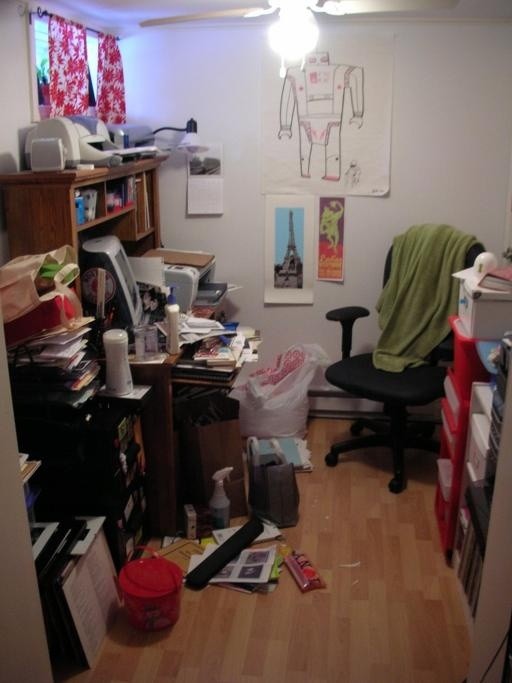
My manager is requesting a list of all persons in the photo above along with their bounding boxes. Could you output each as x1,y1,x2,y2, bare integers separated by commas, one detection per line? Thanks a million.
139,290,162,327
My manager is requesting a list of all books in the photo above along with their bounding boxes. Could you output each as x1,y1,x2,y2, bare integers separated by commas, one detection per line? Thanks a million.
135,172,150,233
174,317,265,381
18,453,41,485
476,265,512,291
216,583,263,593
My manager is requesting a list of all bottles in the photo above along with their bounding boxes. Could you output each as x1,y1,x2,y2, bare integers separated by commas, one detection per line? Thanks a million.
113,127,130,149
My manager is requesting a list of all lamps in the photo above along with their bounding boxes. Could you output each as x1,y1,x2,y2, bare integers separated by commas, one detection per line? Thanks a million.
146,114,212,158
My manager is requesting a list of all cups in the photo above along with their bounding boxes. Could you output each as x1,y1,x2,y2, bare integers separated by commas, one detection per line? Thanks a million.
101,328,133,397
74,196,85,226
133,324,159,362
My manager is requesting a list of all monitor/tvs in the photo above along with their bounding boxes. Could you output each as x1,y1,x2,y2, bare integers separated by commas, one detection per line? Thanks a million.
82,235,143,343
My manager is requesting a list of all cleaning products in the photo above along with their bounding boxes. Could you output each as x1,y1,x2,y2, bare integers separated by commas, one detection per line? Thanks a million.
208,466,236,530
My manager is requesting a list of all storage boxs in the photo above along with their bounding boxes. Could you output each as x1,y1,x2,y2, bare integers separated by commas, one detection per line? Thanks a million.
430,250,510,620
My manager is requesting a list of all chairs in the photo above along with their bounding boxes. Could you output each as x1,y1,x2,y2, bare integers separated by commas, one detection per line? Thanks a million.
319,222,488,497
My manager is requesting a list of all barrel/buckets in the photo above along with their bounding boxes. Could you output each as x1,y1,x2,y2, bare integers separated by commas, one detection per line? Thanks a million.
116,557,183,634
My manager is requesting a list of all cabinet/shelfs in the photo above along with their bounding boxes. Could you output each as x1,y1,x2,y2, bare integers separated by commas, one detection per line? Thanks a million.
1,143,273,579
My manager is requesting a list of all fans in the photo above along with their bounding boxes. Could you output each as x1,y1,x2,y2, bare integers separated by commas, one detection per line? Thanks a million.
133,0,466,32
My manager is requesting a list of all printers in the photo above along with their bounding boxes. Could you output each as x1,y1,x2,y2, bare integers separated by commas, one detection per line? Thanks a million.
155,248,228,313
25,115,156,170
106,124,155,161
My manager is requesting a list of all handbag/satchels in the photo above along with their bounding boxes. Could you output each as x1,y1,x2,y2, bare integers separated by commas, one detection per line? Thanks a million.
247,436,300,529
0,244,84,331
